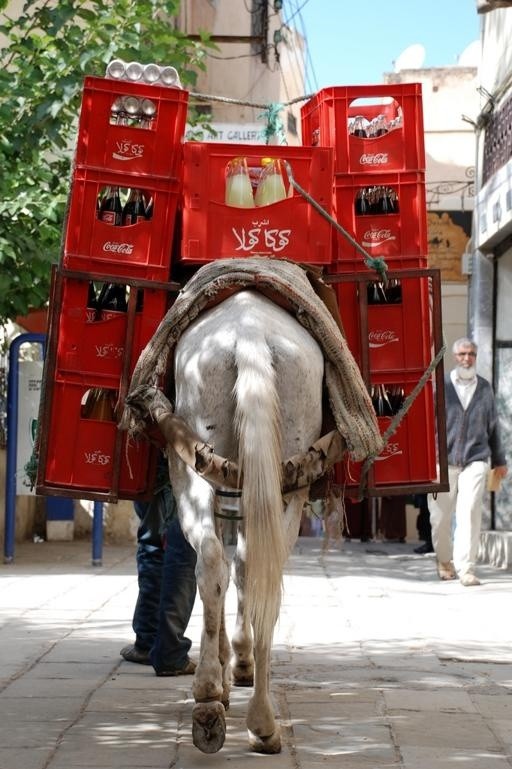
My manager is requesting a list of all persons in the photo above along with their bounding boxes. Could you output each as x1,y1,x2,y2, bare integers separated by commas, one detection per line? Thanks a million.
425,335,509,587
118,442,198,678
407,494,434,554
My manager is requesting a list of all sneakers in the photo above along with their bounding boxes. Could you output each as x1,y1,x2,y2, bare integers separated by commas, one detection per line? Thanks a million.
459,572,481,586
119,644,153,666
156,655,197,677
413,542,433,553
437,560,456,580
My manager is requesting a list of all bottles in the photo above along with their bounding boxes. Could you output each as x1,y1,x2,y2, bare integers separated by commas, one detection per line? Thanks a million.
85,279,144,323
224,154,294,209
95,185,155,227
356,187,398,216
369,384,406,416
109,110,153,130
310,114,404,146
79,388,119,423
356,279,402,306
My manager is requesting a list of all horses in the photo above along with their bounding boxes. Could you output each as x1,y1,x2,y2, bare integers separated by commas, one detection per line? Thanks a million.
166,290,324,755
